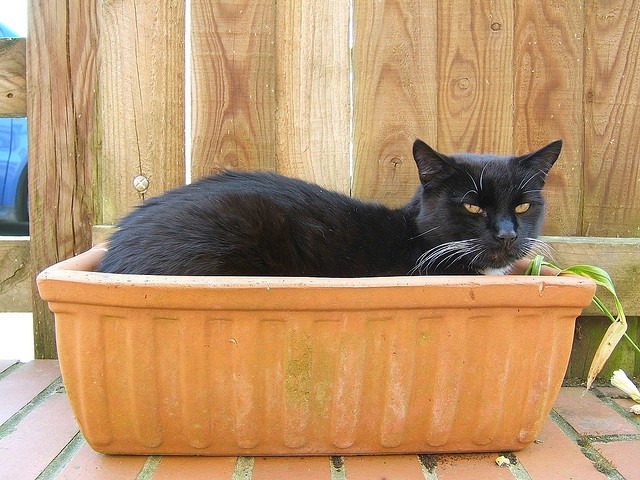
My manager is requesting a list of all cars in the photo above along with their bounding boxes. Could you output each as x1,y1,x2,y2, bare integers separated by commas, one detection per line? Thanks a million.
0,23,26,225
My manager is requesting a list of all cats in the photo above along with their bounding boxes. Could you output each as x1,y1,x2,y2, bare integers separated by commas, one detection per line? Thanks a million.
94,138,563,277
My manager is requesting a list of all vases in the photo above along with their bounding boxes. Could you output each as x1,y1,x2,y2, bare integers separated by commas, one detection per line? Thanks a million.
37,243,596,457
